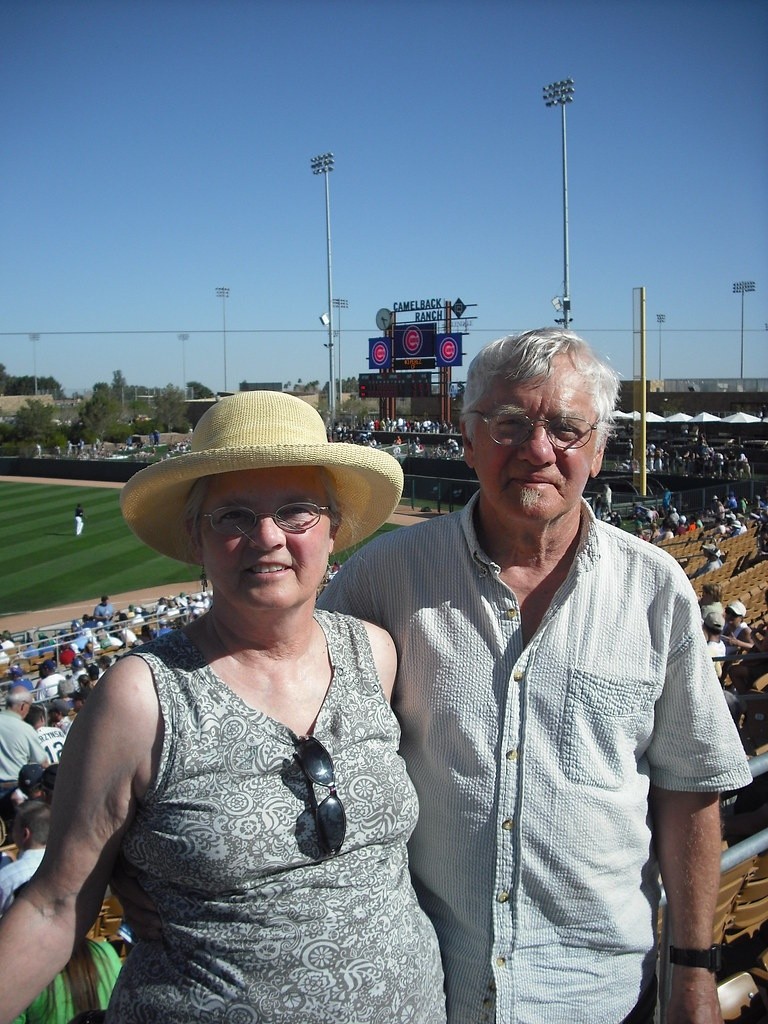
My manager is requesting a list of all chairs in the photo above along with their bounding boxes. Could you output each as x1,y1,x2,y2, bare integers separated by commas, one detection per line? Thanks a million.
653,803,768,1024
88,894,129,967
0,616,171,677
656,516,768,681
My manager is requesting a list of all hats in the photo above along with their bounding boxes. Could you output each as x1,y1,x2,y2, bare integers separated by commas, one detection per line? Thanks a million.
591,431,768,557
703,612,726,631
117,388,404,564
726,600,746,618
0,590,214,794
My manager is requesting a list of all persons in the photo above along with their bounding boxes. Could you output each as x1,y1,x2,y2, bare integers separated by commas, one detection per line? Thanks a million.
698,581,755,680
0,763,136,1024
319,330,752,1024
689,492,768,554
75,503,86,538
626,433,751,480
34,430,194,462
0,389,447,1024
594,483,687,543
0,597,213,803
688,543,724,579
326,416,459,458
315,561,340,597
721,664,768,729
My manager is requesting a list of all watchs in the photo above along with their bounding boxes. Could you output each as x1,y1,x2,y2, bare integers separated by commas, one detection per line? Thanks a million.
670,944,722,971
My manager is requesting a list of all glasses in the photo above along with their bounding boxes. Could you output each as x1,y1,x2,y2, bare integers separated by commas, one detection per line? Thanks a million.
461,408,603,448
200,499,330,538
725,608,741,619
292,737,346,854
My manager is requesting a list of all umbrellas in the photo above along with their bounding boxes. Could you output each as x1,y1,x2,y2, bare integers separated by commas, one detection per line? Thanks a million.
722,412,761,423
646,411,665,423
663,412,693,422
610,409,625,419
687,411,721,422
626,411,641,421
